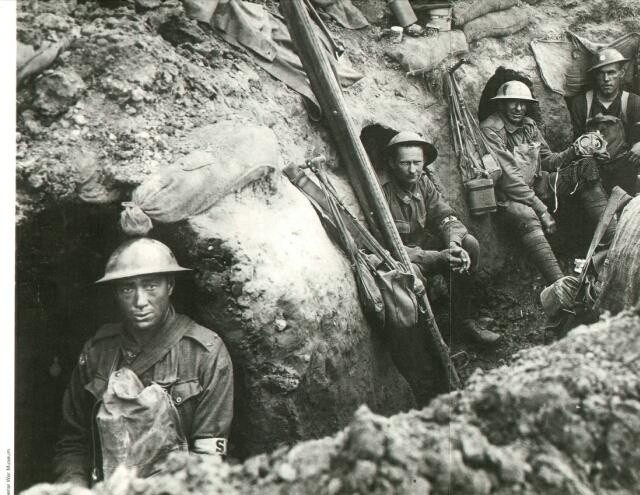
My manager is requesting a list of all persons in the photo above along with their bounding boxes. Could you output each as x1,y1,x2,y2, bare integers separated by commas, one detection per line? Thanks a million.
480,81,617,284
49,237,235,489
568,49,640,196
373,130,500,345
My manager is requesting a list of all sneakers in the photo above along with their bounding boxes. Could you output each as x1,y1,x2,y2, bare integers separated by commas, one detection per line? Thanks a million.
454,318,502,347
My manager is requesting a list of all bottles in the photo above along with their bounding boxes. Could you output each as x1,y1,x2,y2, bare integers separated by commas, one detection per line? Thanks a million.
465,171,498,216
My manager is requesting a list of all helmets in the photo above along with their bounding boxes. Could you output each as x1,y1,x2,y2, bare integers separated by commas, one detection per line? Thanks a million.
586,47,631,73
380,131,437,169
489,80,539,102
93,237,193,284
